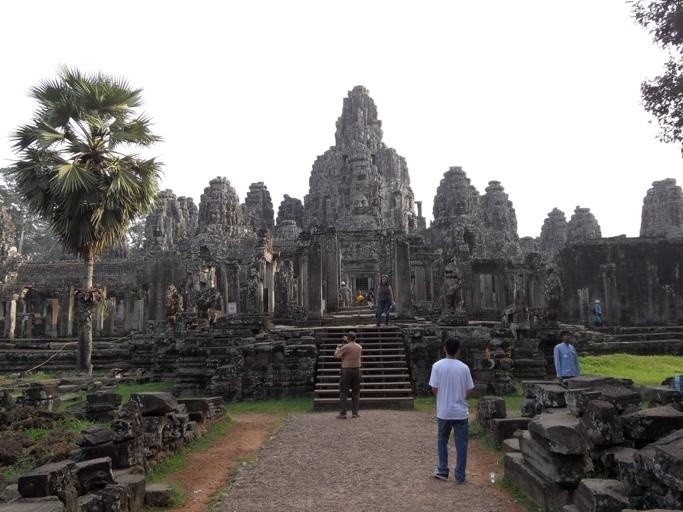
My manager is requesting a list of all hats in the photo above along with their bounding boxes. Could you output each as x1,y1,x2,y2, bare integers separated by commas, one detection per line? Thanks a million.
594,299,600,303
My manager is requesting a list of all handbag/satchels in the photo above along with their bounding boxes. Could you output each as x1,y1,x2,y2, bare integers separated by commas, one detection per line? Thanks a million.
593,316,601,324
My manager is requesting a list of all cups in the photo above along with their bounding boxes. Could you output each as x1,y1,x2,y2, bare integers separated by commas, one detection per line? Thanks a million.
673,369,680,391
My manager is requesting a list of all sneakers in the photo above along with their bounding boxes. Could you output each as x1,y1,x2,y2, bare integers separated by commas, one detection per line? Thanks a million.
353,414,362,418
432,473,448,480
336,413,347,419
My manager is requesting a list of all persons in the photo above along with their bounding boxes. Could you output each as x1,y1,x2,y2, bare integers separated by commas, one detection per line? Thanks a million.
366,288,375,308
373,273,395,329
332,331,363,420
552,328,582,387
591,298,603,327
349,192,370,216
355,288,364,306
340,281,351,306
427,336,476,485
